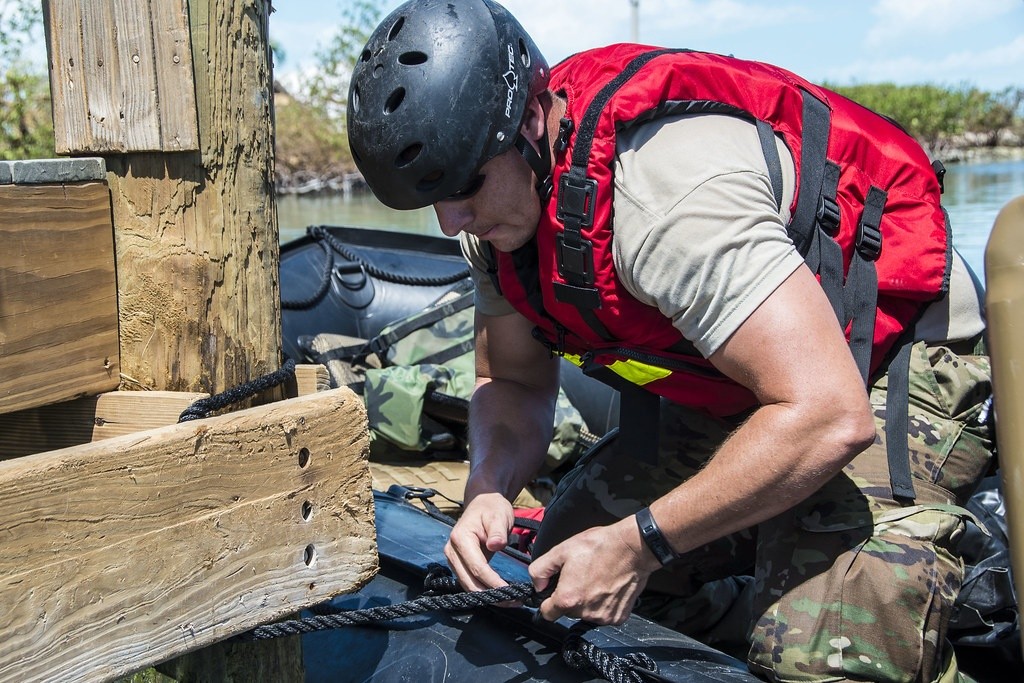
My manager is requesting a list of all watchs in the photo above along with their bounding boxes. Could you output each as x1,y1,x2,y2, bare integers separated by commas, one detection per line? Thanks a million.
634,506,681,567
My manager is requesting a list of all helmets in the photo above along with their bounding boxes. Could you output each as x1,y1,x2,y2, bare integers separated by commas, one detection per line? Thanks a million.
344,0,552,212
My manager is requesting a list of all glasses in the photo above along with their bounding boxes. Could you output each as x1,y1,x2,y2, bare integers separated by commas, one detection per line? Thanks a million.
443,172,490,202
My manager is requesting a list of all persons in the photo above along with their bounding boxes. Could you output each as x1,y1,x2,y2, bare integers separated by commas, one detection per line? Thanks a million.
350,0,953,627
